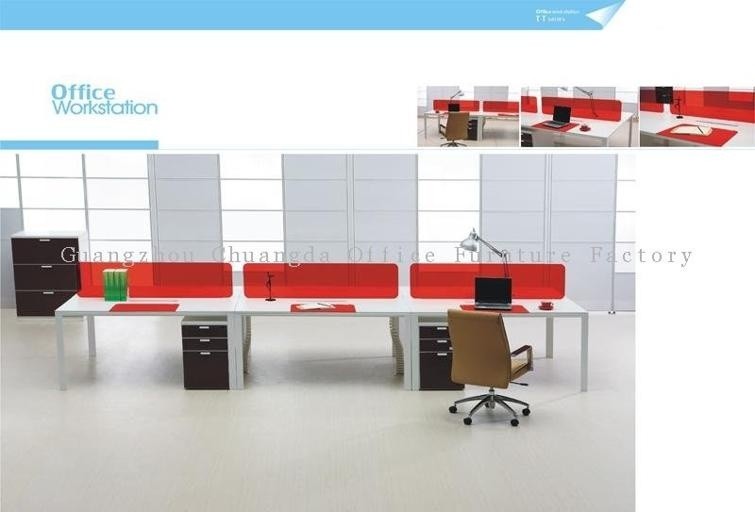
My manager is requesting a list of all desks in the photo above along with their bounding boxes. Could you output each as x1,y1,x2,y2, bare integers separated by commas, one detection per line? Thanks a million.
423,99,480,142
479,100,519,142
641,89,755,147
521,96,553,147
522,97,640,147
641,88,668,146
50,260,591,395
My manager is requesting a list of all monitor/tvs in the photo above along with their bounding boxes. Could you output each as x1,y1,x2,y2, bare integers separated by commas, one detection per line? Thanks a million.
656,87,673,103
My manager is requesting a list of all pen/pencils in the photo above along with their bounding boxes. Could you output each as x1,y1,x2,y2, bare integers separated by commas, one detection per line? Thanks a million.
697,125,703,133
317,303,327,306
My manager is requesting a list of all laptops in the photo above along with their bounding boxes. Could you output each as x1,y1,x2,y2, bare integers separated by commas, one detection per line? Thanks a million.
543,105,571,128
474,277,513,311
447,103,459,113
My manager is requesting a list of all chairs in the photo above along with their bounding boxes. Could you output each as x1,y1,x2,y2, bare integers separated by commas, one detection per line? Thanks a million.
438,107,473,148
443,308,535,427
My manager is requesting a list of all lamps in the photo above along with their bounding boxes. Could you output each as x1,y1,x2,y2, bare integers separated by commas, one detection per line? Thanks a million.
458,227,513,311
558,87,598,121
447,88,465,105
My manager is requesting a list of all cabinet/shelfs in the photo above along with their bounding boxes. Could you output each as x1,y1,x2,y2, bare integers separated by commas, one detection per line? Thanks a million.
522,131,532,147
464,119,477,141
182,313,230,391
11,226,88,315
416,316,465,392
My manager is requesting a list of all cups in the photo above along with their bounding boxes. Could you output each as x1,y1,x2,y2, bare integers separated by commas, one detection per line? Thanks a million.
581,124,588,129
540,302,554,308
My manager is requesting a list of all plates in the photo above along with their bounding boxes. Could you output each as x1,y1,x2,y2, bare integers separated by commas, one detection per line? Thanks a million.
539,305,553,311
580,127,591,132
